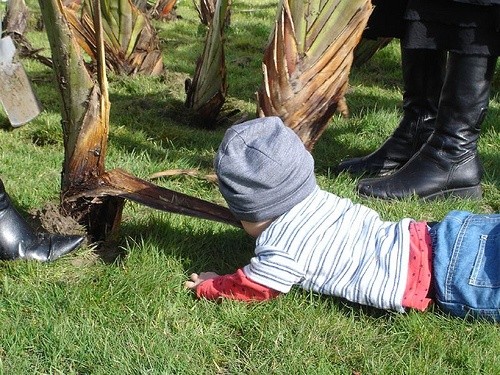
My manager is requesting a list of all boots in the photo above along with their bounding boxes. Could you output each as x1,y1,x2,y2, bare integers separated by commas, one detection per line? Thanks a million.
332,48,495,204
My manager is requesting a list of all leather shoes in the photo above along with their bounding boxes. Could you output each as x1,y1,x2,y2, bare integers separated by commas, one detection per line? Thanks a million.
0,179,85,264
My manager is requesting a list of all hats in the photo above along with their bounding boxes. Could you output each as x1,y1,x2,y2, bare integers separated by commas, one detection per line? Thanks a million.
214,115,317,222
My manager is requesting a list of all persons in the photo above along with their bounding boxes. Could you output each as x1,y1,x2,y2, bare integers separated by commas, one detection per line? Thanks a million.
0,178,85,264
183,117,500,322
335,0,500,205
